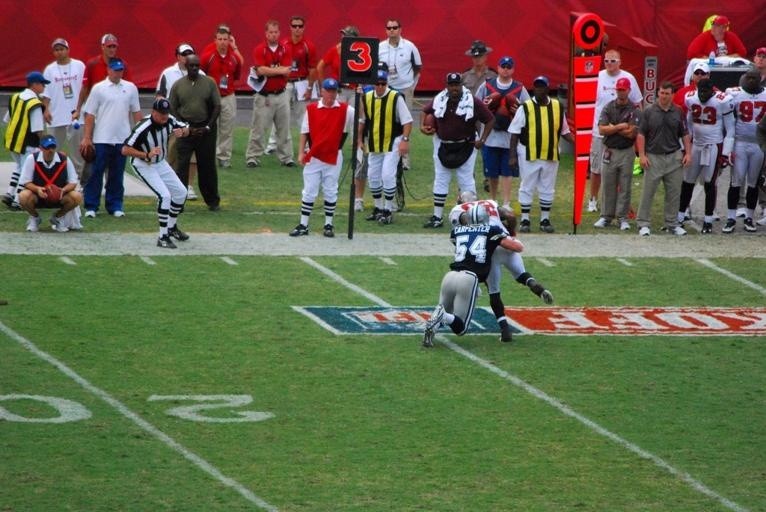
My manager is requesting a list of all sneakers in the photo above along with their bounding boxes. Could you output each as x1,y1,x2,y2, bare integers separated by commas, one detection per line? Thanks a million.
168,224,189,241
218,159,233,167
85,210,96,218
423,336,434,347
289,224,309,235
588,198,766,236
324,224,334,237
286,162,297,167
423,215,444,228
27,215,41,232
540,219,554,233
113,211,125,218
186,185,198,200
354,199,364,212
158,234,177,248
209,200,220,211
425,304,446,336
2,193,14,205
381,209,392,224
48,214,69,232
498,329,512,343
530,283,553,304
366,207,382,220
11,202,24,211
519,220,530,232
248,162,257,167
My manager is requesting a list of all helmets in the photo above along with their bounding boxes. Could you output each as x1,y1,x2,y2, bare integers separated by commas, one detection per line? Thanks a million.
466,206,489,224
457,191,479,204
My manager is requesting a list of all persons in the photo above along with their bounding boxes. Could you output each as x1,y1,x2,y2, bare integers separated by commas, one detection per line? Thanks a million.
588,12,766,236
288,62,414,237
422,192,526,349
449,191,556,344
1,33,221,248
203,16,421,167
419,37,573,233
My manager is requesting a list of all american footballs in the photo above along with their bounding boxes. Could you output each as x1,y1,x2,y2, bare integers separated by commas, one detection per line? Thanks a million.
46,185,60,203
424,113,436,126
488,92,502,110
505,94,520,110
82,144,96,162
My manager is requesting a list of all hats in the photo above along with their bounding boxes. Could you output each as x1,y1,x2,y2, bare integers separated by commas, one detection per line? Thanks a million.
447,73,462,82
52,38,68,48
378,71,387,81
26,72,51,84
322,78,338,89
101,34,119,47
498,57,514,66
756,48,766,55
153,98,171,113
107,58,125,71
464,40,492,56
533,76,549,87
616,78,630,91
40,135,56,148
710,15,729,25
694,63,710,73
378,62,388,70
340,26,358,37
176,44,195,56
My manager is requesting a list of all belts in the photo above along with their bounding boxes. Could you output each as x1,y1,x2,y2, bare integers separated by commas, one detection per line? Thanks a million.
288,77,306,82
260,89,284,96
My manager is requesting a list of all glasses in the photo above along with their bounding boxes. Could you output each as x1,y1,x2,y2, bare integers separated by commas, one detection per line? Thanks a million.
695,72,706,75
375,82,387,86
387,26,399,29
44,144,55,150
502,64,512,69
291,25,304,28
604,59,621,64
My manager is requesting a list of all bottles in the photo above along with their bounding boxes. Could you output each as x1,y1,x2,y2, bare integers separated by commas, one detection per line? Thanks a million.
709,51,716,68
